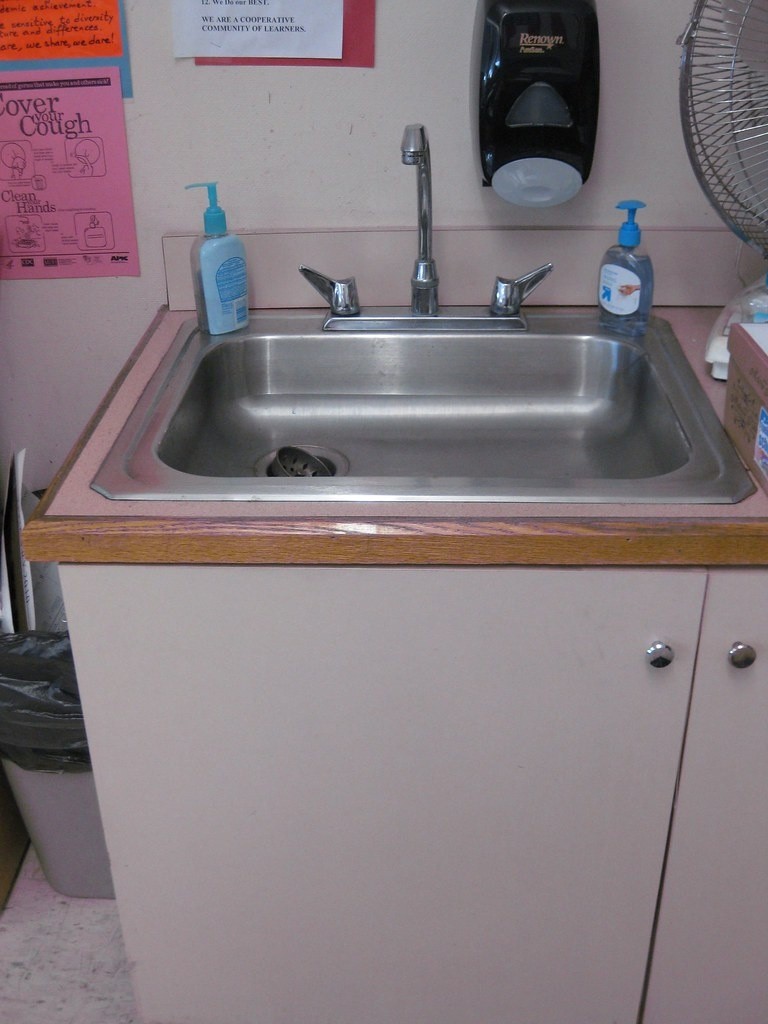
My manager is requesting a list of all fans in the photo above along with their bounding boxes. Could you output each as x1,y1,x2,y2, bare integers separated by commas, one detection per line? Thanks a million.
677,1,768,378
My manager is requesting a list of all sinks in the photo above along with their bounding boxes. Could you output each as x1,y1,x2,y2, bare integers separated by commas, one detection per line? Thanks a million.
88,336,759,504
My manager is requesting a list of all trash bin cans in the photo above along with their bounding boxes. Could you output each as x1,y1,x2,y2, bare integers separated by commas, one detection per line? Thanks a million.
0,490,116,899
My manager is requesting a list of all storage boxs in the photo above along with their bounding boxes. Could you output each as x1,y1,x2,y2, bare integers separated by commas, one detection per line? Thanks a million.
724,322,768,497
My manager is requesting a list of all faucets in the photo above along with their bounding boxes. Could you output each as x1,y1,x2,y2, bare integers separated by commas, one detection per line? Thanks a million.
399,123,440,314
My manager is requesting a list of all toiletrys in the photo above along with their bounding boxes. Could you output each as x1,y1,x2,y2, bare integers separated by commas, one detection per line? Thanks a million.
597,199,654,324
184,181,250,335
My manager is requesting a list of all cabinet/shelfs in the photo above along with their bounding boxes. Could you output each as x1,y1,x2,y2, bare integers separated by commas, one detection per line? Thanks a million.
59,565,768,1024
